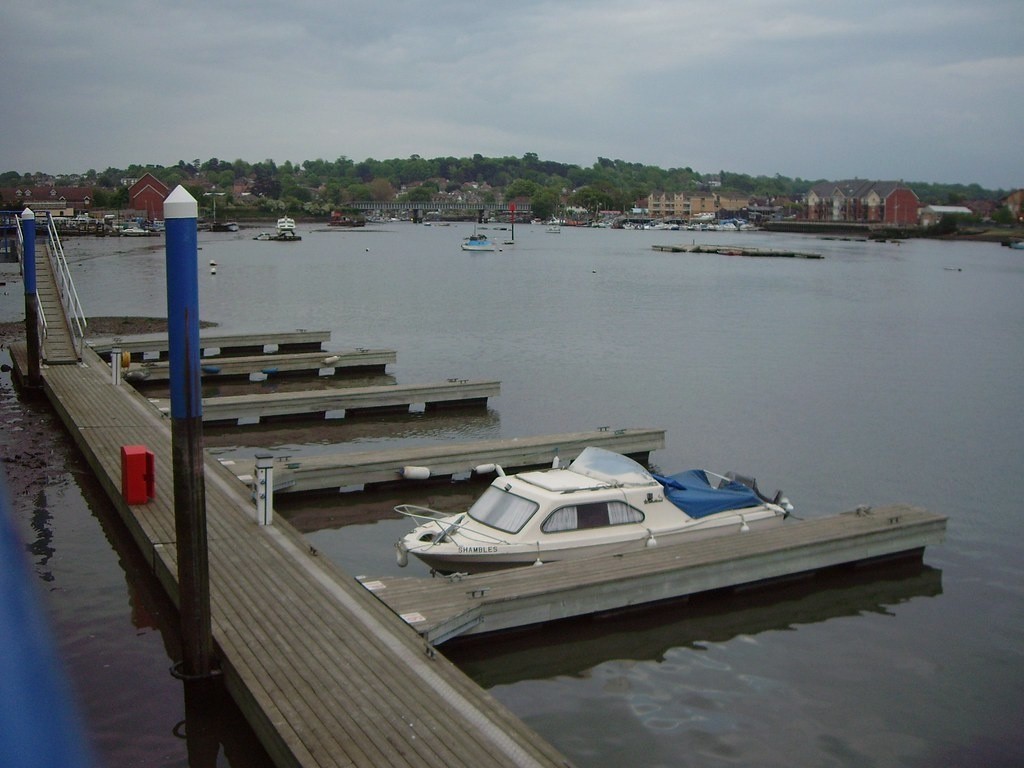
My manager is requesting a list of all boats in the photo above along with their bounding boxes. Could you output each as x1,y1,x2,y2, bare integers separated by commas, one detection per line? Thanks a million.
400,443,798,579
461,238,496,251
